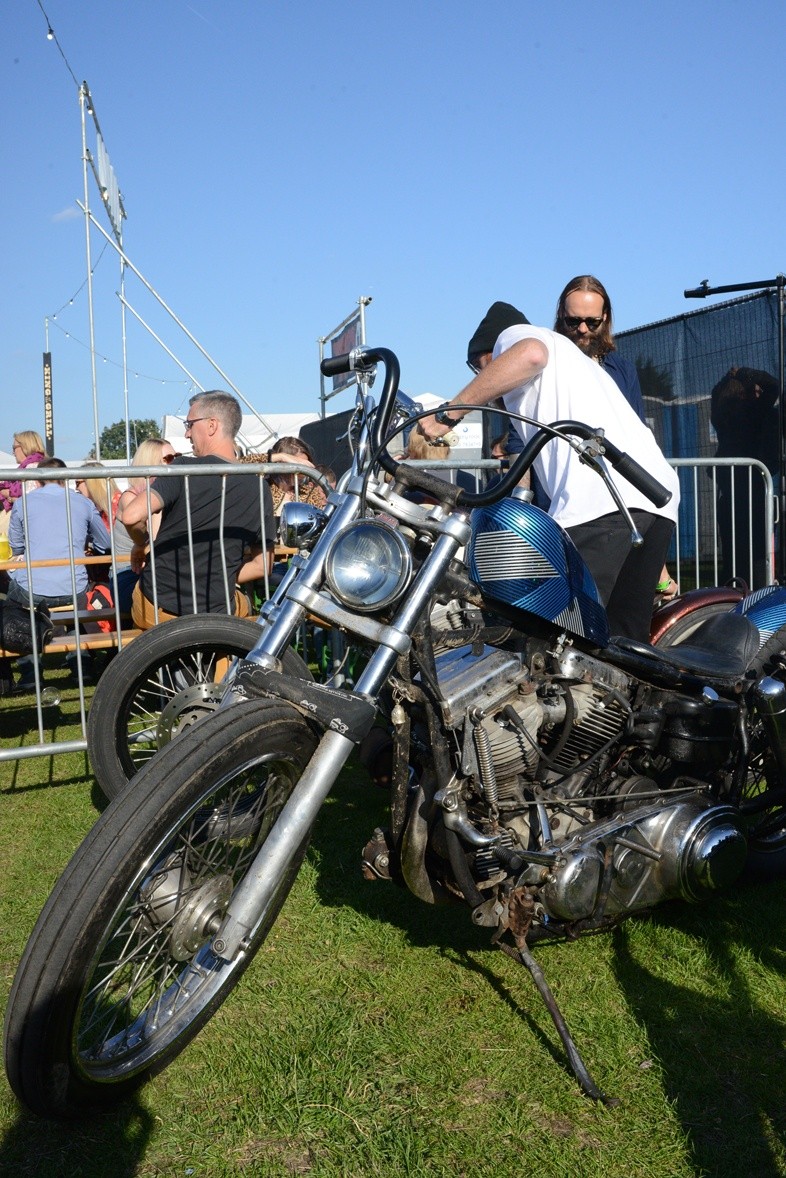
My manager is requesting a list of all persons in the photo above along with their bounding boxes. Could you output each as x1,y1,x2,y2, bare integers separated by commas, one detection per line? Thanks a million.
244,421,521,673
0,430,182,696
707,367,786,591
415,301,681,641
506,274,645,514
122,390,280,684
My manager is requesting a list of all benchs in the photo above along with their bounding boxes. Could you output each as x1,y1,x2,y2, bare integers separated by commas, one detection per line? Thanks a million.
0,608,143,698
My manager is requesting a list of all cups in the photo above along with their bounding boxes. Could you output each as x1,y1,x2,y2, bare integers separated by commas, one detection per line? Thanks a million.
0,538,12,562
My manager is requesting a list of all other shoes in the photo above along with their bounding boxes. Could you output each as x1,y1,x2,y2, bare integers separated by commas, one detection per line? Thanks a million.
69,669,92,681
16,672,44,688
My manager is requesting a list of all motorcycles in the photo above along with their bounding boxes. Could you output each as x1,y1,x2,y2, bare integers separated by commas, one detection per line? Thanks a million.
2,343,786,1132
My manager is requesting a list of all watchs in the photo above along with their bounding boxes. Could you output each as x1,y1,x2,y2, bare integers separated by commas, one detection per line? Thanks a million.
436,402,465,427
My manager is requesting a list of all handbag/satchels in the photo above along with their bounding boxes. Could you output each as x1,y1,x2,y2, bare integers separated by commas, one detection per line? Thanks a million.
0,598,53,652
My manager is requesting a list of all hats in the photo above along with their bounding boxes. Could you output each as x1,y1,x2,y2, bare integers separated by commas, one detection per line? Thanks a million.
468,302,531,361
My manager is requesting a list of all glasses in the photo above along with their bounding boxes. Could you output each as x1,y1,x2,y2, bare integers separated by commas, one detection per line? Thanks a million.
563,312,603,330
76,481,85,487
183,417,211,431
163,453,182,464
12,445,21,451
